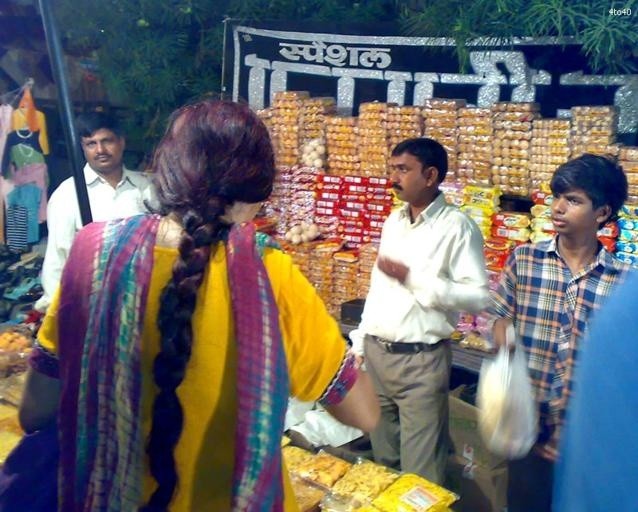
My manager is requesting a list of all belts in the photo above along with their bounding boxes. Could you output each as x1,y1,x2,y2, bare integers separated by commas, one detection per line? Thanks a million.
372,336,438,353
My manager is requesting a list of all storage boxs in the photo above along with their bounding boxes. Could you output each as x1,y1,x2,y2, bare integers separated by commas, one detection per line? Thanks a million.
443,382,510,511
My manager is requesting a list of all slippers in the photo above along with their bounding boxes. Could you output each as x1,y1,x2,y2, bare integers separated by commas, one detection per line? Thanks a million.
0,244,43,335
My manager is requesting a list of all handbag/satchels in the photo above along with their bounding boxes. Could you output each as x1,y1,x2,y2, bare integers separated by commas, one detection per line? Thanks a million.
3,429,59,512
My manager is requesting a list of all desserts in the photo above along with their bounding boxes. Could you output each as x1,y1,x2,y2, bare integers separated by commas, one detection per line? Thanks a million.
282,434,460,512
247,91,637,354
0,323,37,464
